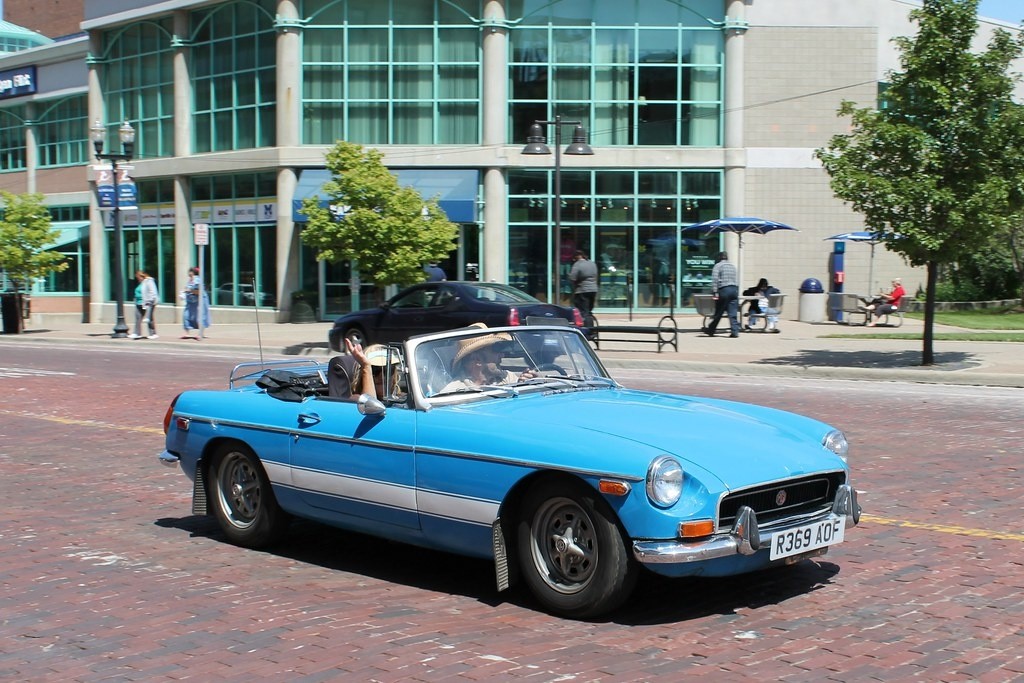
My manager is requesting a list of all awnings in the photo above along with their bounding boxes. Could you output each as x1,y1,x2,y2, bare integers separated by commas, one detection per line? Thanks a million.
291,168,479,225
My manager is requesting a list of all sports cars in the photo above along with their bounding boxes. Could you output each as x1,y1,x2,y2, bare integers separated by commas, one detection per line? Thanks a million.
159,324,864,624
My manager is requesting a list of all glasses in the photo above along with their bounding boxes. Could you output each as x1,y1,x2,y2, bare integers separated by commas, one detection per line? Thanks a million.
481,344,505,353
370,365,395,376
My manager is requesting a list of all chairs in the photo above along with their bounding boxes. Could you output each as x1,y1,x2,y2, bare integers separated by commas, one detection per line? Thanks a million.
827,290,913,328
327,355,368,400
694,292,788,333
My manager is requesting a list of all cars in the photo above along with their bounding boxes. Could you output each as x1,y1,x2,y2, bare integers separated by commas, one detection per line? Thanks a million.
329,277,589,366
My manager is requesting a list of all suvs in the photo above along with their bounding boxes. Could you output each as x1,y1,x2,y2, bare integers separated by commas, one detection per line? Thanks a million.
207,283,274,308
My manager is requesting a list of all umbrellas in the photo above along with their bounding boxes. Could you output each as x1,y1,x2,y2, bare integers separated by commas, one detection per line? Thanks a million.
823,231,905,296
681,218,799,296
645,232,705,247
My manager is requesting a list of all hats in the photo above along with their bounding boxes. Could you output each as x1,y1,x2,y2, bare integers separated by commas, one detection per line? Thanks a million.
356,345,402,368
450,322,513,374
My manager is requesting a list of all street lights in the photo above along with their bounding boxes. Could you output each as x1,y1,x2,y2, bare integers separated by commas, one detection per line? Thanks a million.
90,115,138,339
521,116,594,307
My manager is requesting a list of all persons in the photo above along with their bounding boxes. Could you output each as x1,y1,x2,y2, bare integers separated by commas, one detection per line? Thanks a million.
866,278,905,326
423,261,447,283
744,279,781,332
178,268,209,339
701,252,740,336
639,256,655,306
346,336,410,405
570,250,598,340
126,271,159,340
441,322,537,395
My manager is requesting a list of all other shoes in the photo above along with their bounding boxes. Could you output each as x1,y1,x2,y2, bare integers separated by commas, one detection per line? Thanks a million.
866,319,876,327
853,320,864,325
727,332,739,338
146,334,159,340
180,335,191,341
702,326,716,337
745,322,761,330
763,324,776,332
193,335,201,342
126,334,143,339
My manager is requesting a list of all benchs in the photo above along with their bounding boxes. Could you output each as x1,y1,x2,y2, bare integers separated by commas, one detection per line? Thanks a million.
579,313,680,354
